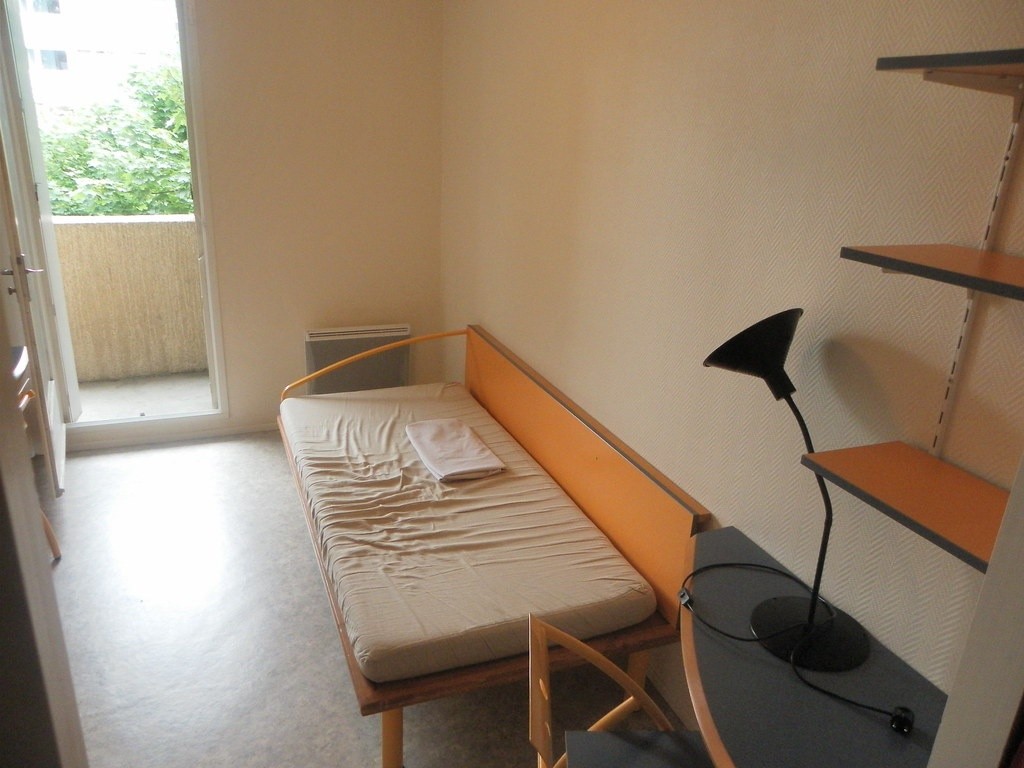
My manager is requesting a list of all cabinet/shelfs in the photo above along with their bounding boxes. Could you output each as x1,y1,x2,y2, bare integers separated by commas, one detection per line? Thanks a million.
678,48,1024,768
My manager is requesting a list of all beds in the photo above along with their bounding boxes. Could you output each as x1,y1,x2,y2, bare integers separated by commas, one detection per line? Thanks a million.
276,322,712,768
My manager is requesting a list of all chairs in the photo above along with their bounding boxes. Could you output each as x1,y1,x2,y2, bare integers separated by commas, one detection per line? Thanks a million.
529,612,716,768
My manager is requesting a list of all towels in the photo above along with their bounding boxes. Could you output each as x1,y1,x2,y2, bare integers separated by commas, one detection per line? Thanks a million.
405,418,508,484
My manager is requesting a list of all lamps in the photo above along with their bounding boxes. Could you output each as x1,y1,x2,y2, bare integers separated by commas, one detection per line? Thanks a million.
701,306,875,672
